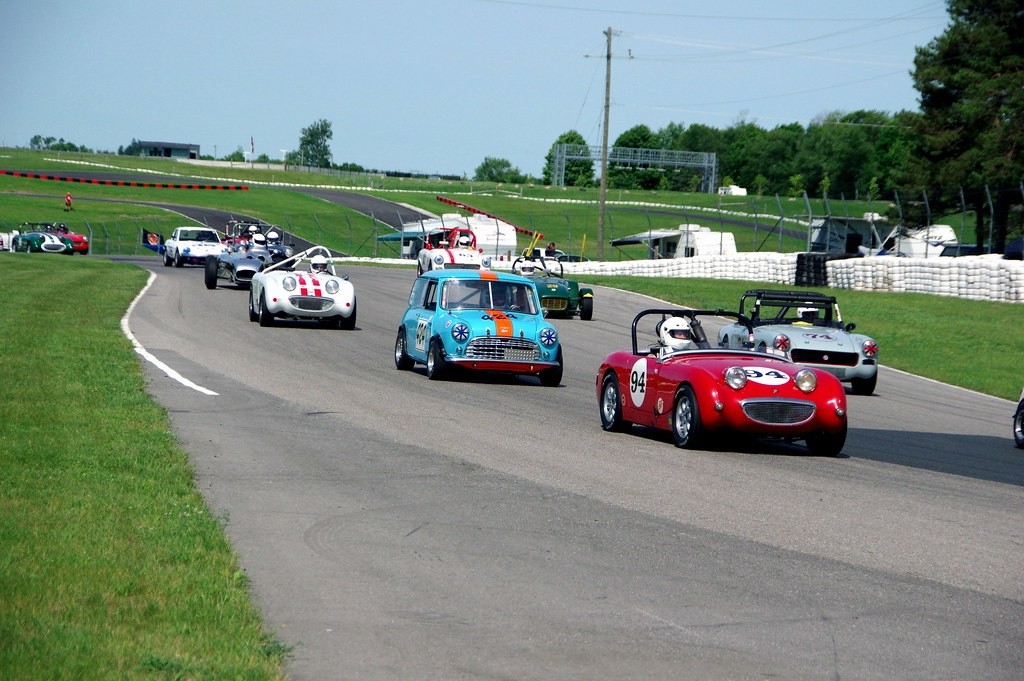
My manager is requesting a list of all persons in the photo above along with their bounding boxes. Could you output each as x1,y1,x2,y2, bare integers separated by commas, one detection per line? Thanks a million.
545,243,555,257
267,231,279,244
248,226,257,236
520,261,535,277
310,255,328,274
57,223,67,234
252,233,265,247
658,317,692,355
457,236,472,248
797,302,819,319
65,191,72,211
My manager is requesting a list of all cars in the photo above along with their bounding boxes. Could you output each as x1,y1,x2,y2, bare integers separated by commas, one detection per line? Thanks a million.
481,255,594,320
393,266,565,392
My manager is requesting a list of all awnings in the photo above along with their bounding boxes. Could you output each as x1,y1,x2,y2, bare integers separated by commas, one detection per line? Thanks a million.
610,232,683,260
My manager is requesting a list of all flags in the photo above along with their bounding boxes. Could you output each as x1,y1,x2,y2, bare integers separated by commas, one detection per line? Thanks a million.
141,227,164,246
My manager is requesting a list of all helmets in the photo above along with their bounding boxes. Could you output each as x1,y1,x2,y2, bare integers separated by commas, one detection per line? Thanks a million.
482,286,511,310
520,260,536,275
797,300,819,321
251,233,268,248
660,317,692,351
310,254,328,273
248,225,259,236
458,236,471,249
58,223,66,231
267,231,280,244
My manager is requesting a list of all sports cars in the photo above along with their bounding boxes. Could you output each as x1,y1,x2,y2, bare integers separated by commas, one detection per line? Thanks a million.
416,226,491,278
163,226,230,266
717,290,879,396
206,222,297,289
39,222,89,256
593,306,848,459
248,244,358,331
11,221,74,255
